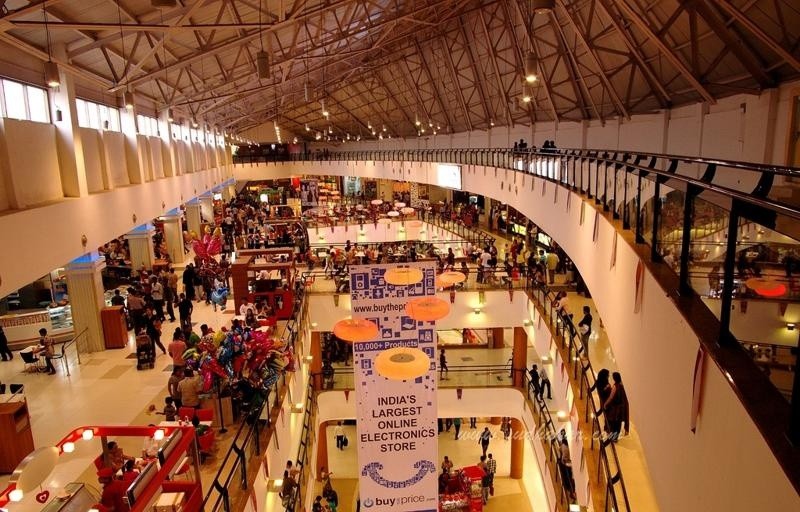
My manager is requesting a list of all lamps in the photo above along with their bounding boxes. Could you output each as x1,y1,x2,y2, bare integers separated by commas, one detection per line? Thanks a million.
40,1,541,149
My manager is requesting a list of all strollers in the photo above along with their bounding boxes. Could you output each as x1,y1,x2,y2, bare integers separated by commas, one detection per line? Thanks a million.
135,332,155,370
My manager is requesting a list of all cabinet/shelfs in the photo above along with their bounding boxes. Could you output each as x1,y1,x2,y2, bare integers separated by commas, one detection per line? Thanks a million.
1,181,308,512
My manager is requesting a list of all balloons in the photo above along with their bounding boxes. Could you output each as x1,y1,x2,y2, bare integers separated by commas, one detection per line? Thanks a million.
185,326,295,405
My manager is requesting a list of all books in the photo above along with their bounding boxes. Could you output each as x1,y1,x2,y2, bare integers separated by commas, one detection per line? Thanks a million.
191,224,224,260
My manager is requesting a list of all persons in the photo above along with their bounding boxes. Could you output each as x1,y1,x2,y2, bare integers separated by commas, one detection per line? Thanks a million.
603,372,630,441
0,325,13,360
89,177,573,512
513,138,557,159
598,369,617,433
578,306,592,359
307,148,329,160
38,327,56,375
